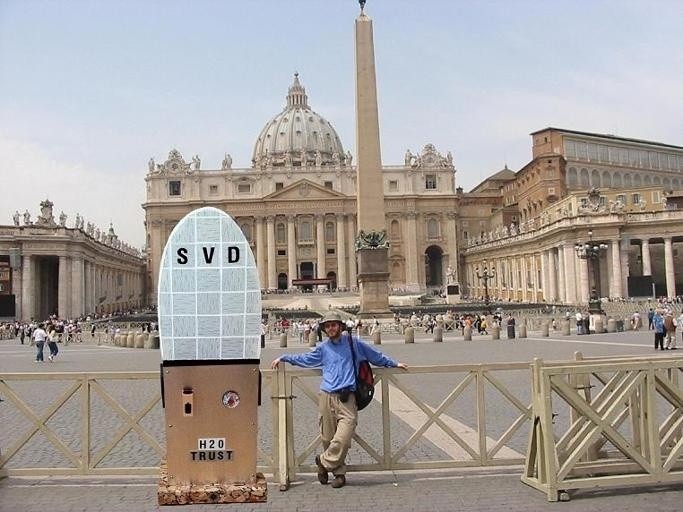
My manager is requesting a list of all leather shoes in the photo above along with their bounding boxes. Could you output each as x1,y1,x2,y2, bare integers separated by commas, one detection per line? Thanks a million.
316,454,329,484
332,475,346,488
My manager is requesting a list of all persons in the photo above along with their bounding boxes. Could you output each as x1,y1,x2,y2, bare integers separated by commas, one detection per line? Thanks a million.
15,210,21,226
332,150,340,167
316,150,321,166
405,149,412,165
227,154,232,168
0,302,158,362
346,150,353,166
552,318,556,329
47,212,56,226
24,210,31,225
75,213,141,257
283,151,290,165
271,311,408,488
575,307,589,335
195,155,200,170
60,211,67,225
599,296,683,351
447,151,452,163
300,149,306,163
261,313,515,349
149,159,154,172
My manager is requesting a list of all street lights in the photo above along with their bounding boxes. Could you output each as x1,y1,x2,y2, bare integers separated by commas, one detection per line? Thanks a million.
474,258,498,305
572,226,610,317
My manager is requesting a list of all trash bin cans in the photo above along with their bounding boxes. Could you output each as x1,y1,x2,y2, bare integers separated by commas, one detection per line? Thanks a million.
508,325,515,339
616,320,623,332
577,322,583,334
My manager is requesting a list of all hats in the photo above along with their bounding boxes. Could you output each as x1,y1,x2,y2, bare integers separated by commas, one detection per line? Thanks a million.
318,311,343,325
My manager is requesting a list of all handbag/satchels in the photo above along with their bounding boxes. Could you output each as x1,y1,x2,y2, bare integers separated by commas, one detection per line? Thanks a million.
49,333,60,342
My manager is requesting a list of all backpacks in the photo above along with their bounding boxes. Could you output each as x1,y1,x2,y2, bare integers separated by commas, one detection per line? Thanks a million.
348,332,375,411
